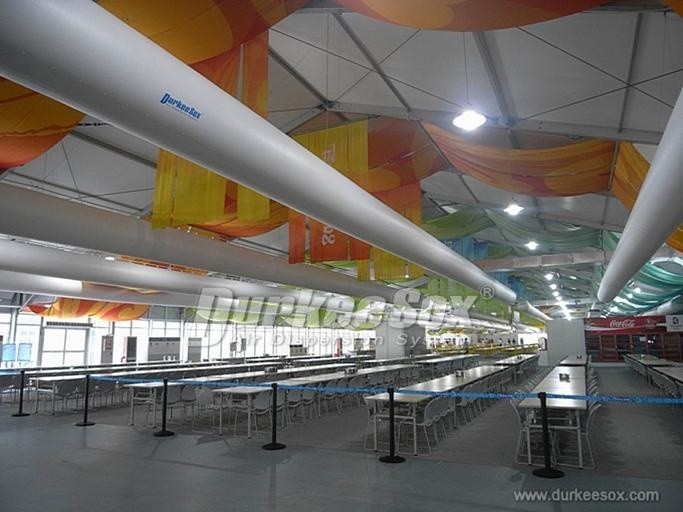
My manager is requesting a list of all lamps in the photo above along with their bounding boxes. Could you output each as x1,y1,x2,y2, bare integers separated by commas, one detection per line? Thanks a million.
452,32,487,131
403,261,410,280
503,133,573,322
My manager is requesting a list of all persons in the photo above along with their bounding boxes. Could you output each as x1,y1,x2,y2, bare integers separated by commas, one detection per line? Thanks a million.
463,338,469,353
481,337,523,346
